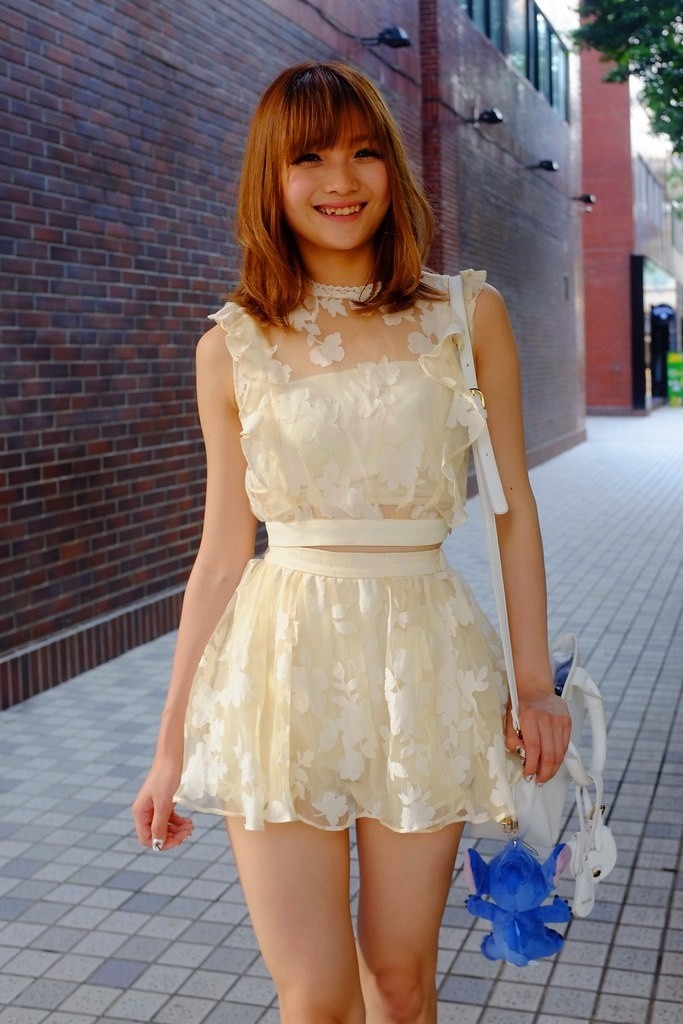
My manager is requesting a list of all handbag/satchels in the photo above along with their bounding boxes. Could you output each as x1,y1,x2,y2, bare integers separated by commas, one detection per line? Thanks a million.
490,628,609,879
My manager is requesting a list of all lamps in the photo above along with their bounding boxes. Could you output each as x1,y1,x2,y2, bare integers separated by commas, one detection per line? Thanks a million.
361,24,411,51
526,159,559,172
571,193,596,203
467,107,504,127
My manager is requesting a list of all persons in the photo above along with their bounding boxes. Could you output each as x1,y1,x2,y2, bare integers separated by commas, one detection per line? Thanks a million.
131,70,571,1024
